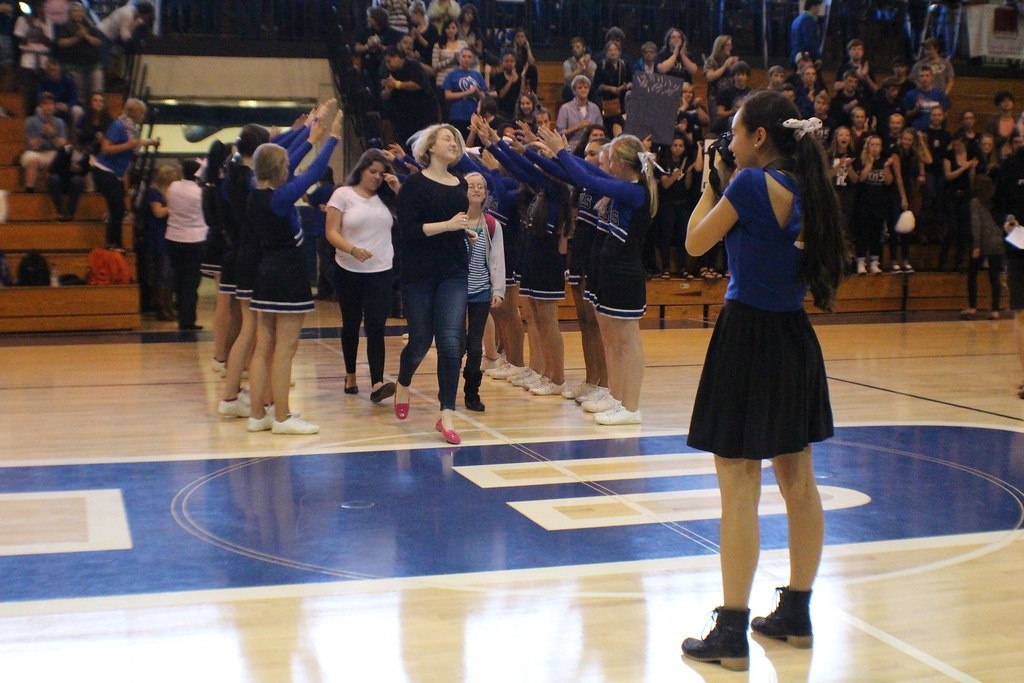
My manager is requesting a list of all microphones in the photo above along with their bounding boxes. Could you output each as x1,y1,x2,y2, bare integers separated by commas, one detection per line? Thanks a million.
1008,215,1017,226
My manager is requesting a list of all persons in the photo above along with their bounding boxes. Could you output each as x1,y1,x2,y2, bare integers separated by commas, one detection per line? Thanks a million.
0,0,1024,444
680,91,853,671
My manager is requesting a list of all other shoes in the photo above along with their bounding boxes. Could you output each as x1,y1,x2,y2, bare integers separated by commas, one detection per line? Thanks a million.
344,376,359,394
212,357,226,372
220,366,248,379
435,419,461,444
644,253,1011,318
395,380,410,420
370,382,395,403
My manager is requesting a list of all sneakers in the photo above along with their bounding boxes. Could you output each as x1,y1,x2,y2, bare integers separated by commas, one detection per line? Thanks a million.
528,380,568,396
263,404,300,418
575,386,610,405
218,398,252,418
512,371,543,387
459,348,525,379
593,402,642,425
272,412,319,436
561,381,596,399
247,413,275,431
237,388,251,407
507,368,537,383
581,395,623,413
523,376,550,391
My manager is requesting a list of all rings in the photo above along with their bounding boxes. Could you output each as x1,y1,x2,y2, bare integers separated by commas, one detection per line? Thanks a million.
463,218,466,222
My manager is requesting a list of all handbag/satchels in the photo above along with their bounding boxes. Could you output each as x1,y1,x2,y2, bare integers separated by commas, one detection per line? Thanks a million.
0,247,129,284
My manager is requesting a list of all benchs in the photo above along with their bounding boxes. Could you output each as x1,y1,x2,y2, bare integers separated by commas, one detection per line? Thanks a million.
0,58,1024,340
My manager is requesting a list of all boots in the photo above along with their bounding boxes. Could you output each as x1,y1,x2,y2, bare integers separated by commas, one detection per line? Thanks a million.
681,606,750,671
464,367,485,412
751,586,814,649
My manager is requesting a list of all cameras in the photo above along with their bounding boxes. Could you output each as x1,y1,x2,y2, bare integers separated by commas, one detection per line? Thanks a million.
708,130,737,169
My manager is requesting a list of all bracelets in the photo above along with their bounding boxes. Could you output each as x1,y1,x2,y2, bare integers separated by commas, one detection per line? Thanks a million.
445,221,450,232
351,246,356,255
